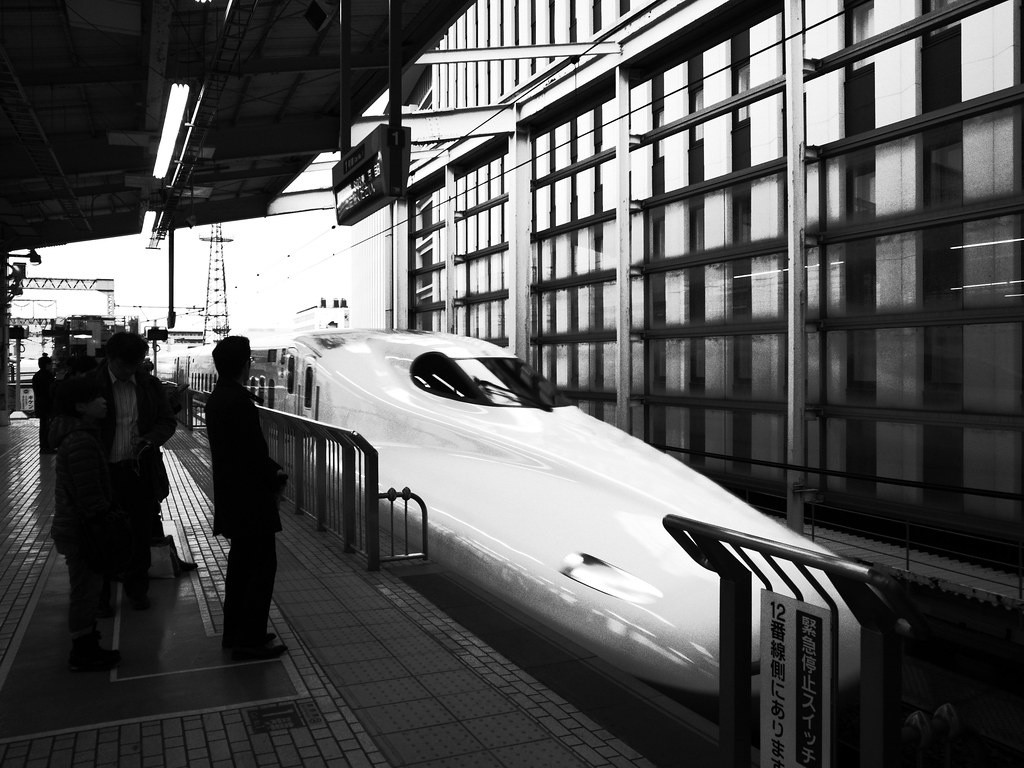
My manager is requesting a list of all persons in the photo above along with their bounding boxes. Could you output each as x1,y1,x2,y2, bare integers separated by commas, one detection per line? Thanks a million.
83,332,177,619
149,386,198,577
204,336,288,663
48,378,121,671
32,357,56,454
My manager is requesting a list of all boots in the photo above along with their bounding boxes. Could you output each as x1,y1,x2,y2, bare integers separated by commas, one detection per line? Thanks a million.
69,631,117,672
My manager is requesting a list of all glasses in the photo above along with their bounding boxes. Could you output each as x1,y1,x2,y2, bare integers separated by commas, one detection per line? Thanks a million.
248,357,255,362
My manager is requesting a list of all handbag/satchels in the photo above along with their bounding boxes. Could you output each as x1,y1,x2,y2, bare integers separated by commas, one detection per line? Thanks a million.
147,546,177,580
75,513,143,578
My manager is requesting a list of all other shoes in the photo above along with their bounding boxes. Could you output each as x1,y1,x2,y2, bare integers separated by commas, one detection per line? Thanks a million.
231,643,288,660
125,588,149,609
94,600,114,618
263,632,279,641
180,561,198,571
40,448,57,454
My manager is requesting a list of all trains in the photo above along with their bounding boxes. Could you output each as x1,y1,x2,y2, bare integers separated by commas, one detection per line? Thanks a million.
150,330,896,709
